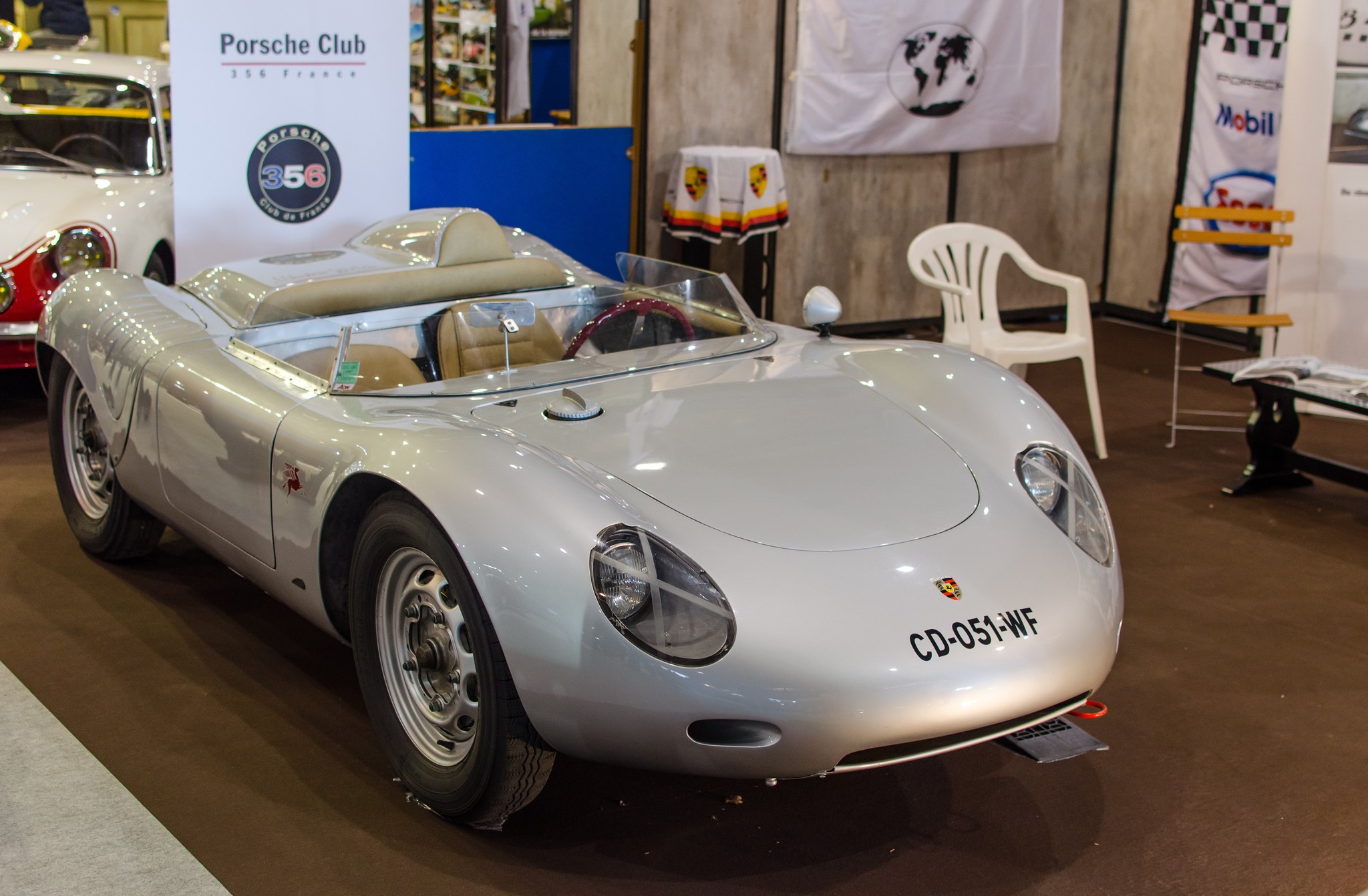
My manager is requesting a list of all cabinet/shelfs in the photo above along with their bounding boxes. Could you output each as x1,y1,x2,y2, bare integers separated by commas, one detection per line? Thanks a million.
406,0,506,126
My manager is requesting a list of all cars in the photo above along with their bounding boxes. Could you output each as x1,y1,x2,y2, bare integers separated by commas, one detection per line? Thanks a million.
0,47,178,405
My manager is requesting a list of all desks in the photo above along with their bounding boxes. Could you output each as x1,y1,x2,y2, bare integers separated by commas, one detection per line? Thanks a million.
1200,356,1367,498
660,145,790,322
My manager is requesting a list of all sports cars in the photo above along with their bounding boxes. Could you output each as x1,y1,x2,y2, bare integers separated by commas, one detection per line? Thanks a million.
30,202,1126,827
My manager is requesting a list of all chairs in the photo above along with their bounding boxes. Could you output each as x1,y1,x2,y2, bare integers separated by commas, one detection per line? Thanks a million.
284,345,427,391
437,299,567,379
908,222,1109,459
1166,206,1297,452
